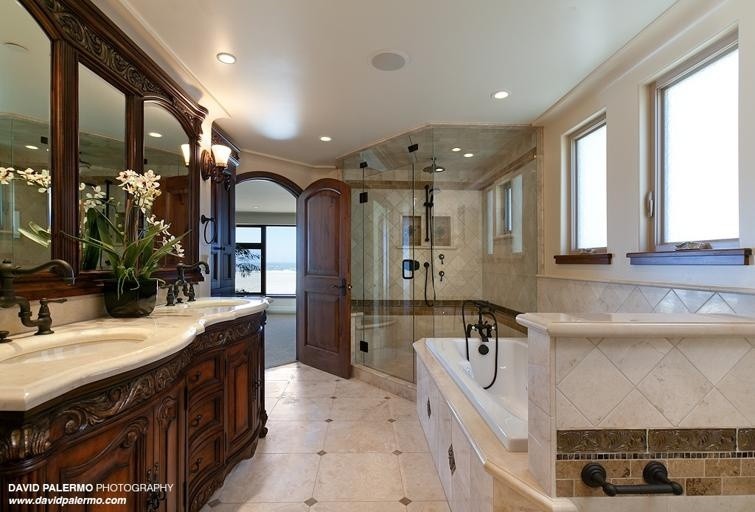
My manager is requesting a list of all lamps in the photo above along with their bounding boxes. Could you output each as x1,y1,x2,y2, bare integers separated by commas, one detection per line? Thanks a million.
200,143,231,183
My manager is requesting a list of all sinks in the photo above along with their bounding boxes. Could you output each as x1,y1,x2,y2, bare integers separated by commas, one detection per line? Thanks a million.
184,301,250,309
0,329,148,365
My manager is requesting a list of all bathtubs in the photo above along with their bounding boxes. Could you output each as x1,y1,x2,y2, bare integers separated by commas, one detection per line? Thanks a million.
426,337,528,452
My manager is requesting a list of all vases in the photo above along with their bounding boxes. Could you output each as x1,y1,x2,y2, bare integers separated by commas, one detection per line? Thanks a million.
105,278,156,317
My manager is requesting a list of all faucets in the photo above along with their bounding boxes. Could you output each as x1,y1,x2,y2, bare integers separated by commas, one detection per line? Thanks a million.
479,330,489,342
176,261,210,282
0,258,75,308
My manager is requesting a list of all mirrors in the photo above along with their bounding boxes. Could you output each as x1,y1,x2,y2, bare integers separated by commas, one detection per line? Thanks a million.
1,0,209,308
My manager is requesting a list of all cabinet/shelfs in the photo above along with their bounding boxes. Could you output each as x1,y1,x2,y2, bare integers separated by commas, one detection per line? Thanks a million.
187,322,224,510
224,312,267,470
0,348,186,512
